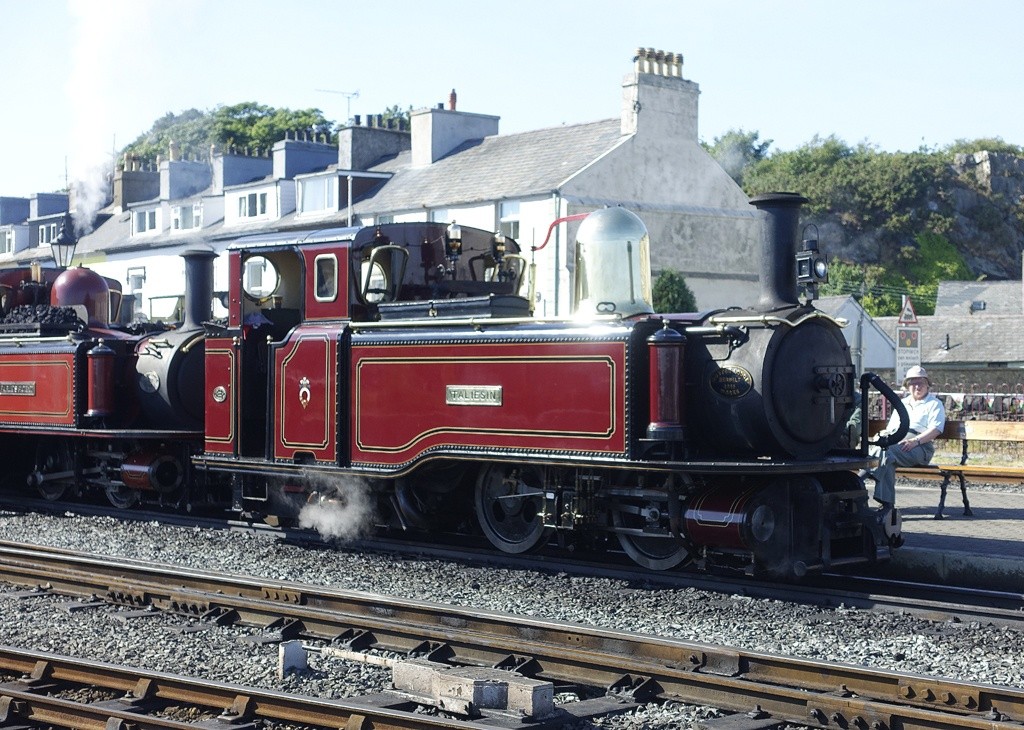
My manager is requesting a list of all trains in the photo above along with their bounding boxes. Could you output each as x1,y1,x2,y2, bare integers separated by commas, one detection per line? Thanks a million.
0,191,908,582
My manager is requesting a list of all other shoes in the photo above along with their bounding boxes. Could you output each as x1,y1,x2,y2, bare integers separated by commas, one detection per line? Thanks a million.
879,505,894,512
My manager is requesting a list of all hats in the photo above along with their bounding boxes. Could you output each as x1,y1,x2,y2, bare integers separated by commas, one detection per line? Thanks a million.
906,366,928,379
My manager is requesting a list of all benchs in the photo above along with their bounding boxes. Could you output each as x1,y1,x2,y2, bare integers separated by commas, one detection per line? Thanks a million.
239,308,300,430
868,418,1024,519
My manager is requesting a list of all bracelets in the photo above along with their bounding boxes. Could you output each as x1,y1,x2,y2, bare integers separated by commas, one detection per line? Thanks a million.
916,437,920,446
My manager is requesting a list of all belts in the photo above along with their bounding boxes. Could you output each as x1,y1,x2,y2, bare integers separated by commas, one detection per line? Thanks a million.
908,428,918,435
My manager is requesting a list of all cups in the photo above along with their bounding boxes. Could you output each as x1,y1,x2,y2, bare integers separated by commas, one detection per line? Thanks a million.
272,295,283,309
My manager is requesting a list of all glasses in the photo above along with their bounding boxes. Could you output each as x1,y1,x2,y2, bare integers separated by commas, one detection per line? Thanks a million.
908,382,926,388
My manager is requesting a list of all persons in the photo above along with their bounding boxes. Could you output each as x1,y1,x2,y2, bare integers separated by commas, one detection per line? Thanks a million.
858,366,945,510
